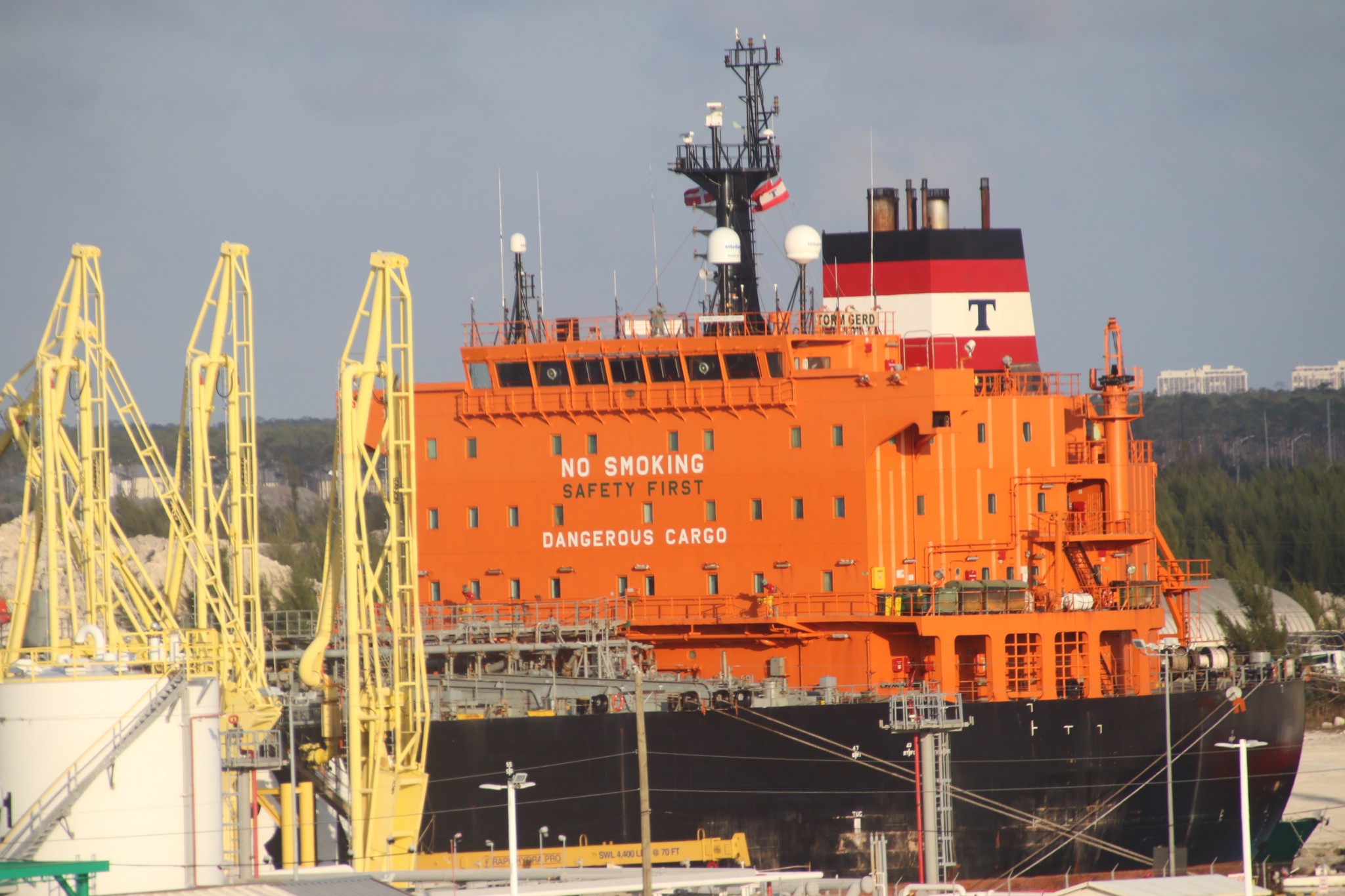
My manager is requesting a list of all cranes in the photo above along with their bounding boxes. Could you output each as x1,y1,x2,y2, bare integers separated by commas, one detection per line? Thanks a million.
4,233,756,868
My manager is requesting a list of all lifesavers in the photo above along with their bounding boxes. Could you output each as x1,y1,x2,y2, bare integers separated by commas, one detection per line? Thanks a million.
611,694,625,711
1102,589,1113,607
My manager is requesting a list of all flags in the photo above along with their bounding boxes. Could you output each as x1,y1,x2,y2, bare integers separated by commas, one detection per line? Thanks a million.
759,176,789,211
751,180,774,213
683,186,715,207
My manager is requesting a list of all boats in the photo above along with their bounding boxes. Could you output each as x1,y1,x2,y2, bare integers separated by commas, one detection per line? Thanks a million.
1275,808,1329,879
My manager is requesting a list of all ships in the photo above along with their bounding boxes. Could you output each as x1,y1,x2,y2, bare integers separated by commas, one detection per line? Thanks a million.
323,29,1309,888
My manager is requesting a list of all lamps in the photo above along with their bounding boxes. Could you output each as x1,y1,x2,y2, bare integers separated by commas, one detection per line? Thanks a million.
964,339,976,358
1126,563,1136,575
858,374,873,387
890,373,904,385
934,568,946,588
